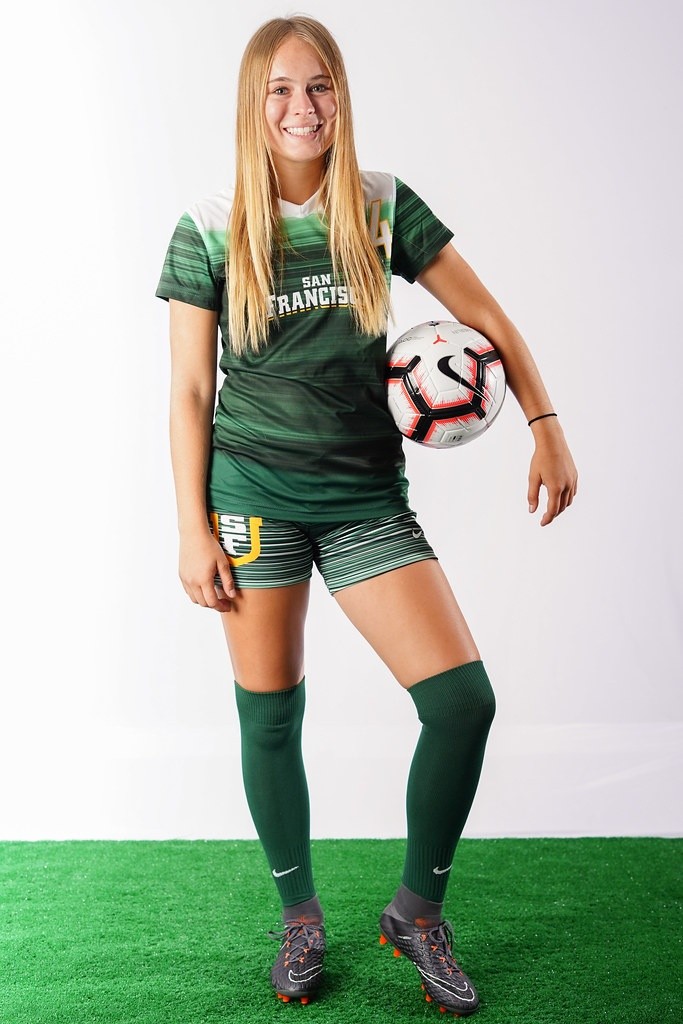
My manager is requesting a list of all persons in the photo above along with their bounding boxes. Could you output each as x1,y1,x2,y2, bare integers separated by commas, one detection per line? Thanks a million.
156,14,578,1018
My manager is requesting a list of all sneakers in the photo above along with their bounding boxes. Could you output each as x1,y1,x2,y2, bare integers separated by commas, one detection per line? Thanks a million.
270,894,326,1004
379,884,479,1018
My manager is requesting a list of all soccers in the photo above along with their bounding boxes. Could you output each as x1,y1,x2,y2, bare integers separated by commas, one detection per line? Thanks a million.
384,320,506,450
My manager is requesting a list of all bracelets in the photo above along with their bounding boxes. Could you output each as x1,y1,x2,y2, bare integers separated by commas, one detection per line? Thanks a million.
528,413,557,426
